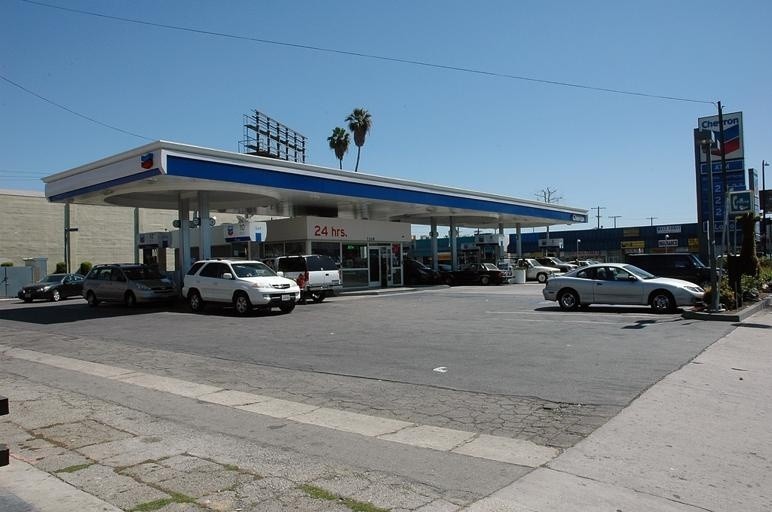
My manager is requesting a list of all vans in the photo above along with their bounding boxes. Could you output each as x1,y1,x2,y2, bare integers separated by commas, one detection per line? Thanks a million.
263,254,345,304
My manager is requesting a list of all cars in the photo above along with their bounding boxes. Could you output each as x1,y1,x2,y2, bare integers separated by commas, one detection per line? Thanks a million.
17,272,86,303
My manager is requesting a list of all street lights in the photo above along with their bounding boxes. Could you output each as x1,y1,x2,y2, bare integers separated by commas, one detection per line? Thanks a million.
761,159,771,259
665,234,670,254
576,238,581,258
64,228,80,273
693,128,727,314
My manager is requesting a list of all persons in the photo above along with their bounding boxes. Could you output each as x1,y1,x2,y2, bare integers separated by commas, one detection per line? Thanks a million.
574,257,580,266
522,258,529,268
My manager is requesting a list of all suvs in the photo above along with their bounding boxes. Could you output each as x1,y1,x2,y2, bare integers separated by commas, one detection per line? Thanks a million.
181,256,302,316
80,262,180,309
